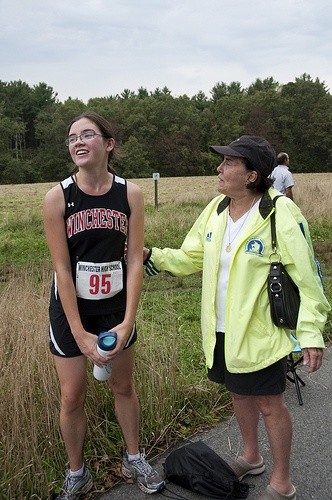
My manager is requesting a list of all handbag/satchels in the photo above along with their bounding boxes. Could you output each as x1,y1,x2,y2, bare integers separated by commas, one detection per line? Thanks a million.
269,195,324,329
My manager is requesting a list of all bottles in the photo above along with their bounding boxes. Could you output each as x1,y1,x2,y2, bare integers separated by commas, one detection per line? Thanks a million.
92,331,118,382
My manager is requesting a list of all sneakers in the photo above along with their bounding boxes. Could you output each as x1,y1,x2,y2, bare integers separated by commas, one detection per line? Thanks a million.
58,464,94,500
229,453,266,480
256,482,296,500
120,451,166,494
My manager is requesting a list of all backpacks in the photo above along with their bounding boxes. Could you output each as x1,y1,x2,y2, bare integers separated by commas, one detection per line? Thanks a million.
159,441,255,500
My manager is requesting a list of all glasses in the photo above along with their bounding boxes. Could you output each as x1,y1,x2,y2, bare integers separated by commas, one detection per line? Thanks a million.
65,131,109,146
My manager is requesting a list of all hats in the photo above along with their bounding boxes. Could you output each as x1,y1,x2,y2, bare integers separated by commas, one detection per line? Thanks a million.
209,135,277,176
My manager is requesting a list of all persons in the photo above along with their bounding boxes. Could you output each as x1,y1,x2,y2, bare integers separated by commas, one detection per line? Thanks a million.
123,135,332,500
42,111,167,500
266,152,294,202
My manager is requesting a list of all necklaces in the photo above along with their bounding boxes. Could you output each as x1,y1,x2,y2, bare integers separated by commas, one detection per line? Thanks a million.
225,193,258,254
